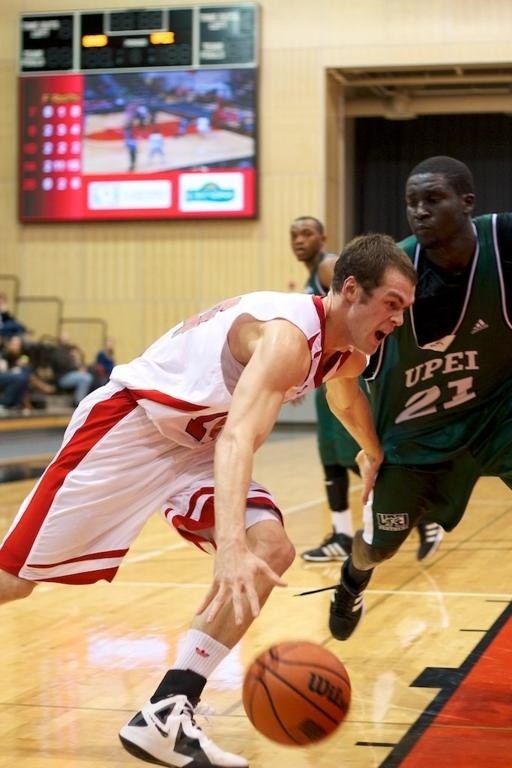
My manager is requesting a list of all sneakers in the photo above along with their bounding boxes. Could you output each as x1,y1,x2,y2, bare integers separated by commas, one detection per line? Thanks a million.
300,533,352,561
417,523,444,560
119,695,248,768
329,556,373,641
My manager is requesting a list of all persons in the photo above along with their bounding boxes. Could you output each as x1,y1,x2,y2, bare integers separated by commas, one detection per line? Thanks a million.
125,100,209,171
0,291,115,416
0,231,419,768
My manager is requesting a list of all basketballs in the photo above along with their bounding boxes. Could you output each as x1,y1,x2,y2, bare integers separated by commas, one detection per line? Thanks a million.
242,640,351,749
